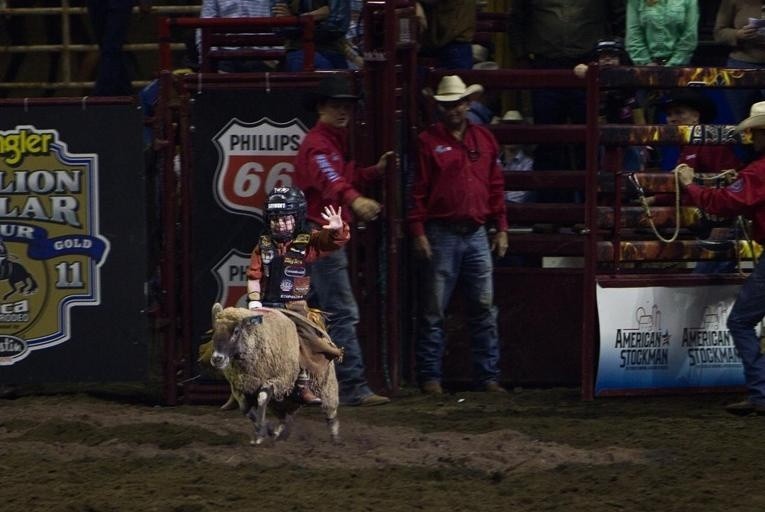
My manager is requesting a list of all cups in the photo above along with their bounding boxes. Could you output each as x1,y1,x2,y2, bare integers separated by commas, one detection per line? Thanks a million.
749,18,764,31
269,3,278,17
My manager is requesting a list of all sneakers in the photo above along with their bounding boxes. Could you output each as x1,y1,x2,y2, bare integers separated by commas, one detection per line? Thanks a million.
285,383,325,406
339,391,392,408
418,378,444,398
219,392,239,410
471,380,510,395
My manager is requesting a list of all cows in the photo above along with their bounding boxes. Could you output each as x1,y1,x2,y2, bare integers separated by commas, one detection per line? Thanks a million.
209,301,340,447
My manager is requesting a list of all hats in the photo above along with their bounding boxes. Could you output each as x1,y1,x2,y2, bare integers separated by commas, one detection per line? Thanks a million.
419,72,486,106
301,71,368,106
731,97,764,133
642,87,720,124
495,109,528,122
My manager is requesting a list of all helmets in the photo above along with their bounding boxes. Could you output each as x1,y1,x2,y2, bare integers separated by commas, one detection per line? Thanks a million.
262,184,309,242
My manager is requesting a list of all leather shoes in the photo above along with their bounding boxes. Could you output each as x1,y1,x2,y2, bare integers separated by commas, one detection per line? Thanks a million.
721,397,765,419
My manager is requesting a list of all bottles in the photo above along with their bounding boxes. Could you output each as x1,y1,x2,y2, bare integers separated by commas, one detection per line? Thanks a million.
597,39,622,69
